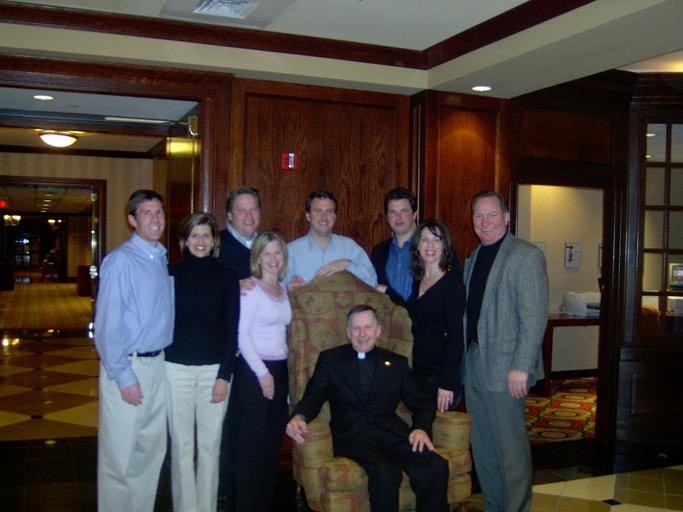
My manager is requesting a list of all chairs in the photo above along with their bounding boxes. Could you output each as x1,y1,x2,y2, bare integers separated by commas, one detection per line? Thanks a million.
285,269,473,511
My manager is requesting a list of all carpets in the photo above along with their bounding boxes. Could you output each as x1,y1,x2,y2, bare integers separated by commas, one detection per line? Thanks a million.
522,377,599,448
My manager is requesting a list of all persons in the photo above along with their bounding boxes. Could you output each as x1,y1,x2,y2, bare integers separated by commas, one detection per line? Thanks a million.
374,218,465,413
163,209,237,512
217,181,263,512
239,229,305,511
366,186,453,306
278,189,379,512
284,305,452,512
461,190,549,512
92,186,178,512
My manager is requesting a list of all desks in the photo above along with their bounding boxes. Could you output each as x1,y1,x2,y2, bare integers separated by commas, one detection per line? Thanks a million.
541,314,602,399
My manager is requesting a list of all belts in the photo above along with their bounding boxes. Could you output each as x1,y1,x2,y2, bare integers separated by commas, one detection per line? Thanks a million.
127,349,162,357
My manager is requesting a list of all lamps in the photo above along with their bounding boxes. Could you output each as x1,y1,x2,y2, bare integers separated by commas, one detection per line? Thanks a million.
38,132,80,147
